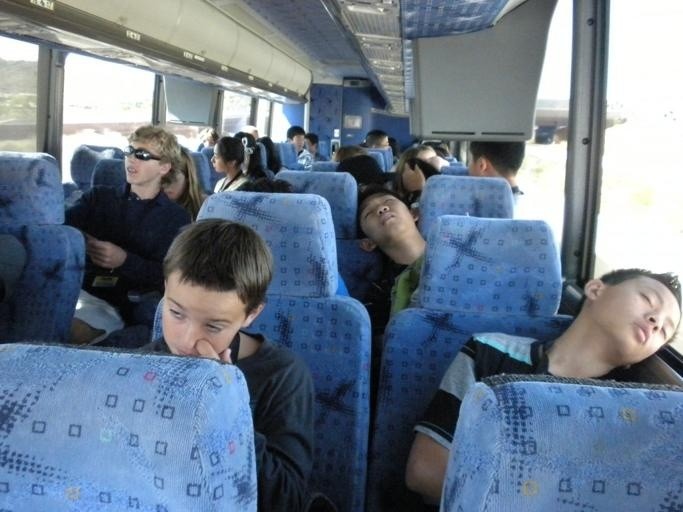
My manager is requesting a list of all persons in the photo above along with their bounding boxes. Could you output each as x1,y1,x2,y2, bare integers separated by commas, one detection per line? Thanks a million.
334,133,525,343
286,124,327,169
199,126,294,195
404,268,682,501
2,125,208,345
138,217,314,511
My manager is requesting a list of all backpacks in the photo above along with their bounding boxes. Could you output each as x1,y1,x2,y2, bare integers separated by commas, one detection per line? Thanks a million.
335,156,388,203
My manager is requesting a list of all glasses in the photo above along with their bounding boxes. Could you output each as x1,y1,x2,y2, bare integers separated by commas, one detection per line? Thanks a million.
124,146,162,161
